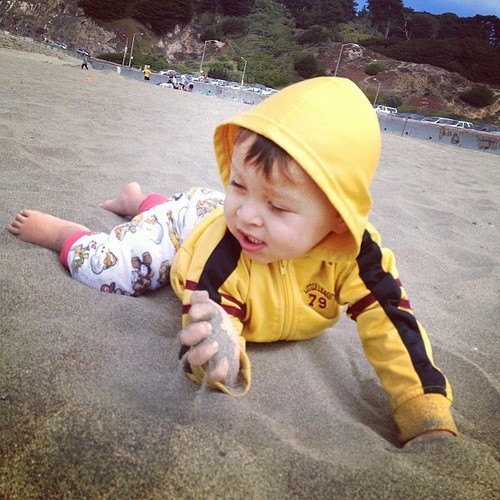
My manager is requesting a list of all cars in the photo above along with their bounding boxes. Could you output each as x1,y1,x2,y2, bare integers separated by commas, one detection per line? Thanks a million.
76,48,92,57
53,41,68,50
163,68,498,133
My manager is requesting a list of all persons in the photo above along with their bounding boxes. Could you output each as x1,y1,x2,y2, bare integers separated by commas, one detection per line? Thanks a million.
4,77,459,450
206,91,213,96
167,74,195,92
81,55,89,70
143,67,151,86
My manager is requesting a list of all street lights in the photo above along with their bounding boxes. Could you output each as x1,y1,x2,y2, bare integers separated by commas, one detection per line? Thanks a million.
333,42,354,77
372,76,381,108
122,32,129,66
198,39,218,74
127,32,145,67
240,55,248,86
